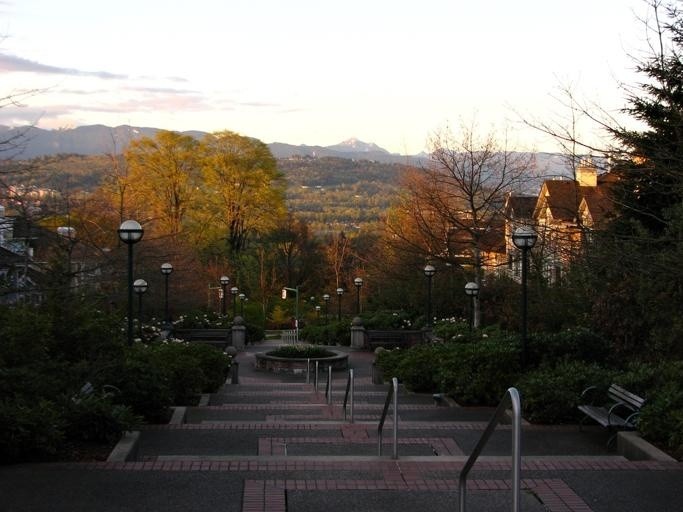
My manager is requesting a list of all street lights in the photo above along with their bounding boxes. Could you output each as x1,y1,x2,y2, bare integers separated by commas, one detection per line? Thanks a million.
118,219,173,347
465,281,479,331
279,284,299,326
315,277,362,327
512,225,538,371
219,274,245,321
423,265,435,326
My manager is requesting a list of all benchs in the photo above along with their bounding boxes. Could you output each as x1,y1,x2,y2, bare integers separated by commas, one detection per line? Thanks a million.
577,384,647,453
